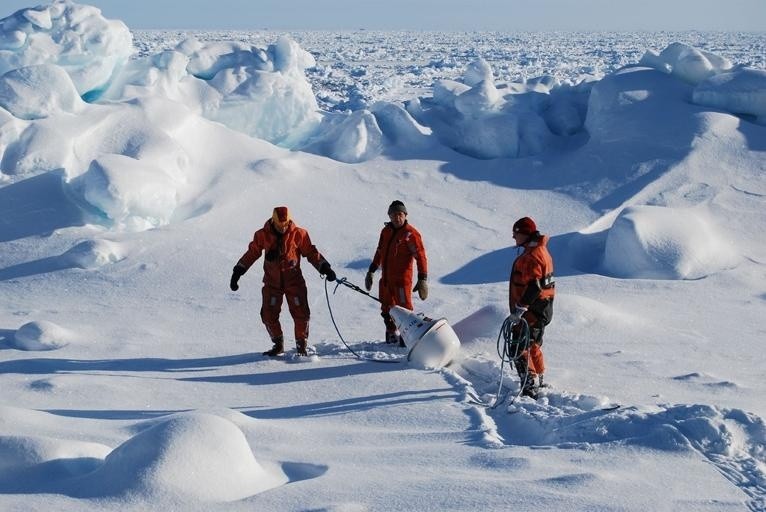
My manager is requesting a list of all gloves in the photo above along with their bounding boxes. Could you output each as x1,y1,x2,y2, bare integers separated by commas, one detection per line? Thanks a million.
365,272,374,291
324,268,336,282
230,272,241,291
413,279,429,301
510,303,528,325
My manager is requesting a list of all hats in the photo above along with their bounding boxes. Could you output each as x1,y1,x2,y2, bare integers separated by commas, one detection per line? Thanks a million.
272,206,289,222
513,216,537,236
388,200,407,215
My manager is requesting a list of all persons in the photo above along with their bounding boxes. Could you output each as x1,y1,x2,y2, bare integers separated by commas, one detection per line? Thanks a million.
365,200,428,347
230,207,336,355
509,217,554,400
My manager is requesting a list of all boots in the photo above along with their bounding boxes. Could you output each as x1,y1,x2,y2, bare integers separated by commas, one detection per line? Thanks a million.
296,336,308,356
262,337,284,356
385,322,405,348
521,375,544,400
